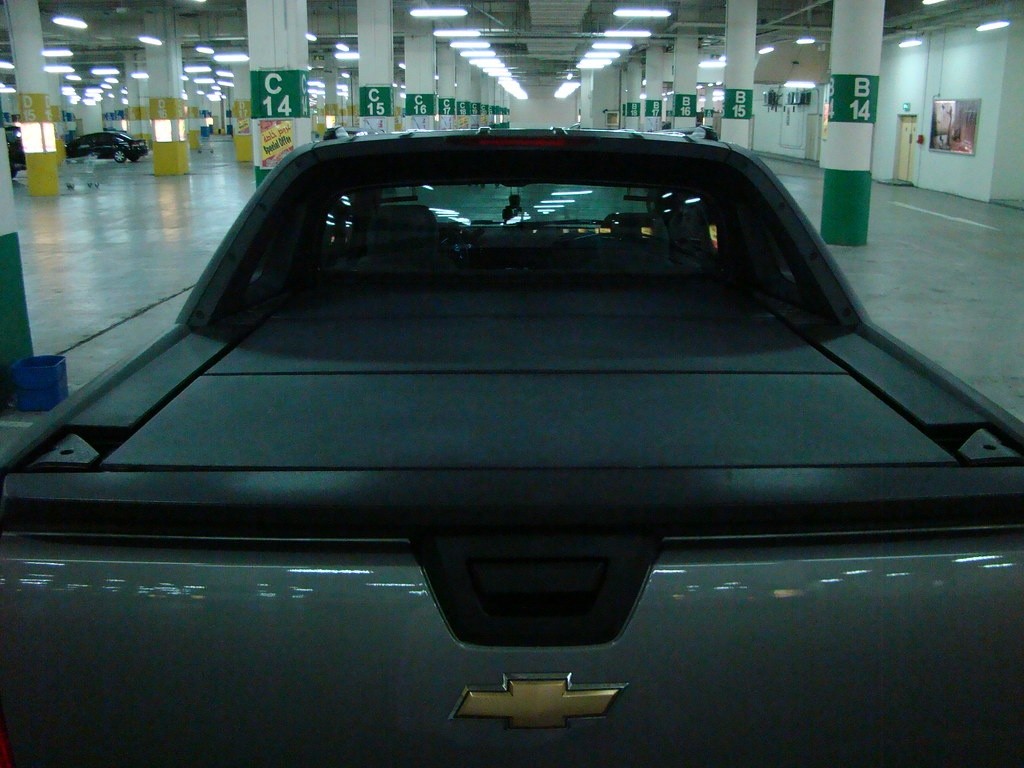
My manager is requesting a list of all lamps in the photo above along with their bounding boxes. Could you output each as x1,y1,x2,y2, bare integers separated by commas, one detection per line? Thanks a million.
0,0,815,107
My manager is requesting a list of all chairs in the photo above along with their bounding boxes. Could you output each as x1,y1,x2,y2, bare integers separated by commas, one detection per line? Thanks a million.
360,201,439,275
597,210,672,276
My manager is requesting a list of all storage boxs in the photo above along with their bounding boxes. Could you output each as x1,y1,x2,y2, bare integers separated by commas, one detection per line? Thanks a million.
11,354,69,412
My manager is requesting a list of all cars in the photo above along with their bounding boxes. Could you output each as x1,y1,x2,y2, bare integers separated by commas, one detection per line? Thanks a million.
0,126,1024,768
66,131,148,164
5,125,27,180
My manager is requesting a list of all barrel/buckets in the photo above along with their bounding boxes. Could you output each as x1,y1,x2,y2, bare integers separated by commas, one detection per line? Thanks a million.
64,134,70,141
11,355,69,412
